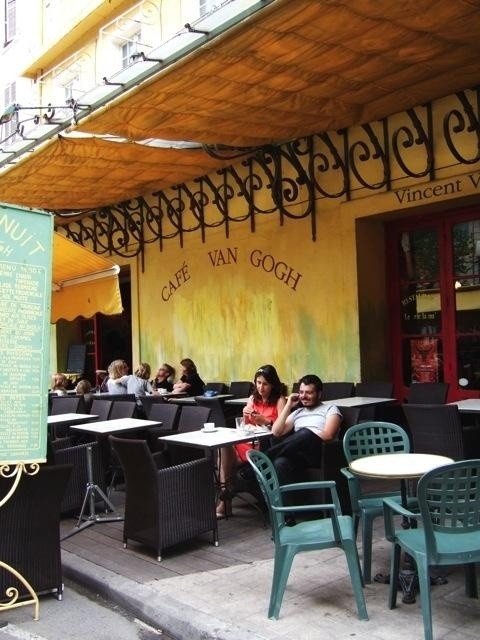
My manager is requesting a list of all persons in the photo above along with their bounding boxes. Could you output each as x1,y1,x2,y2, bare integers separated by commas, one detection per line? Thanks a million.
148,363,175,392
114,362,161,409
106,358,129,395
95,370,110,394
236,374,344,528
49,372,69,395
215,365,288,520
75,380,94,410
171,359,203,395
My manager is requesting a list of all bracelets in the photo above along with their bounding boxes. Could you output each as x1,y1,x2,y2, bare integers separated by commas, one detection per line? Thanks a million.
270,420,273,426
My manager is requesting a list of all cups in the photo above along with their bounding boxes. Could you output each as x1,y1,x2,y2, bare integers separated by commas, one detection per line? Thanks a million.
203,422,216,430
235,417,245,434
158,388,167,394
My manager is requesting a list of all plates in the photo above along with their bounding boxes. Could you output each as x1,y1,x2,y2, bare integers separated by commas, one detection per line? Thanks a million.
201,428,218,433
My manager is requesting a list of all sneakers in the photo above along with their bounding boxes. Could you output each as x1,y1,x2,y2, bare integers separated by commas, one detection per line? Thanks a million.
219,487,235,501
216,499,233,519
236,464,255,481
286,516,297,527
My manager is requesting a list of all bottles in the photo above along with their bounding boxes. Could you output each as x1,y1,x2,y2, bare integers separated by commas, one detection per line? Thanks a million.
96,385,100,395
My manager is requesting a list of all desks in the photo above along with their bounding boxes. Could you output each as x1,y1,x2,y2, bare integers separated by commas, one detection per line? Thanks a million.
350,453,455,605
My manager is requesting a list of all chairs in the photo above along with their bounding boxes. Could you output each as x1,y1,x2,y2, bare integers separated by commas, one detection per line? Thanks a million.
400,380,480,521
48,381,398,543
341,421,438,585
246,448,372,622
382,458,480,639
109,435,221,562
